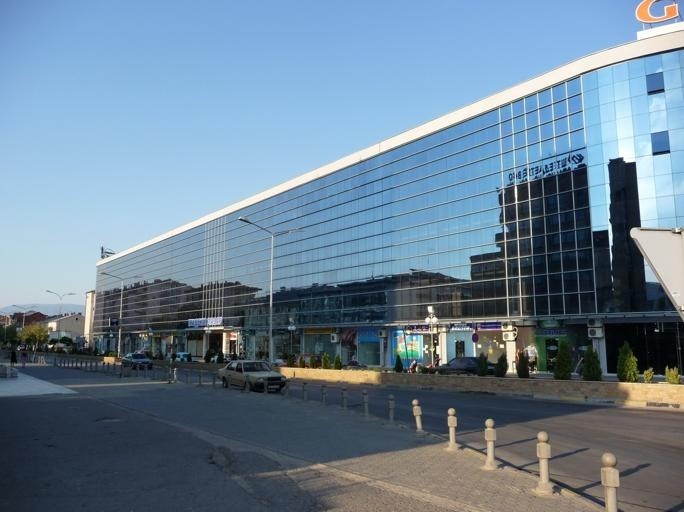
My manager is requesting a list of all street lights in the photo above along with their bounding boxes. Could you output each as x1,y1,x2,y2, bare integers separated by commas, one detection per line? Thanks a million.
204,324,212,350
148,328,154,350
12,304,35,326
239,216,296,363
98,271,142,358
45,289,76,353
425,306,440,368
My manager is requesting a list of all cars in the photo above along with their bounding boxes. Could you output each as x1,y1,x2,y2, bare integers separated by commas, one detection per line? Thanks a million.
217,359,287,393
121,352,153,370
174,352,189,362
17,344,25,351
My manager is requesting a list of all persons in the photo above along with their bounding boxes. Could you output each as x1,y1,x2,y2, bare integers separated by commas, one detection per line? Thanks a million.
21,348,27,368
11,347,17,367
435,354,440,367
